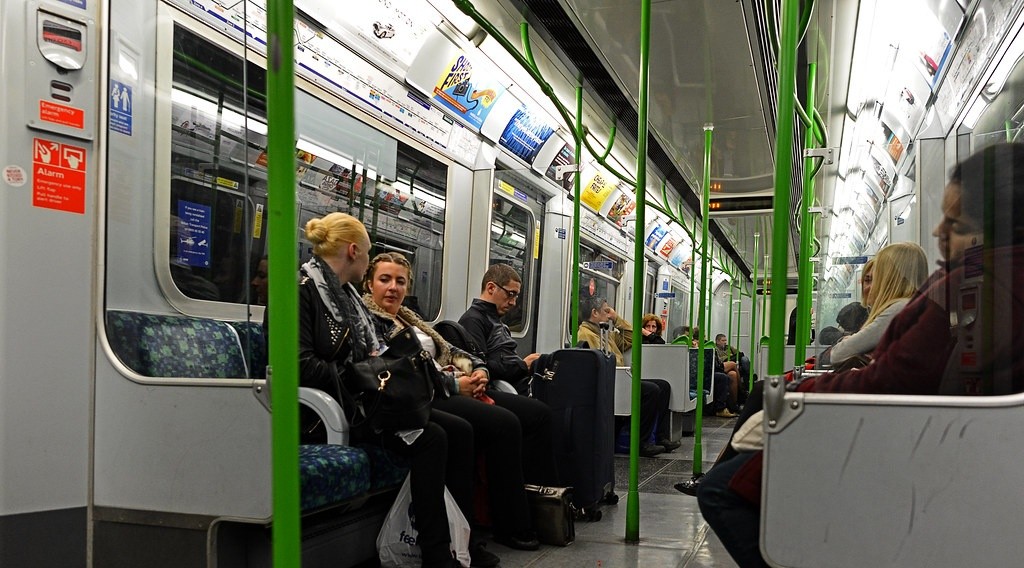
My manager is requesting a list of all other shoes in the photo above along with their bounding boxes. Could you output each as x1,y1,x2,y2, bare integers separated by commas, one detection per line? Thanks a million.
492,528,540,551
659,438,680,452
468,541,500,568
639,444,665,457
674,472,706,496
716,407,736,418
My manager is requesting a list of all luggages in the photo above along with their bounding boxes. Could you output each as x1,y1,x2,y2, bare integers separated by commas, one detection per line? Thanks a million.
530,322,618,522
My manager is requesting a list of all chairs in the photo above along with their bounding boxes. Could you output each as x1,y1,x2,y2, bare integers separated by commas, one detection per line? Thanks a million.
92,310,1024,568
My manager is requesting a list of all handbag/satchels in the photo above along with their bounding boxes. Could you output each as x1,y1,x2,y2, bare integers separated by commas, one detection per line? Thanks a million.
376,470,471,568
344,353,431,430
722,352,744,363
439,365,496,406
730,408,764,452
521,485,577,547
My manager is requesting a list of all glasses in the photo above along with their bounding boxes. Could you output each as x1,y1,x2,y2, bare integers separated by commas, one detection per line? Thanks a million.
494,282,520,300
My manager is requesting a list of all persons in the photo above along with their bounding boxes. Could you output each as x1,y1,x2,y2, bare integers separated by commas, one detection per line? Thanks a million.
262,213,545,567
576,141,1024,564
